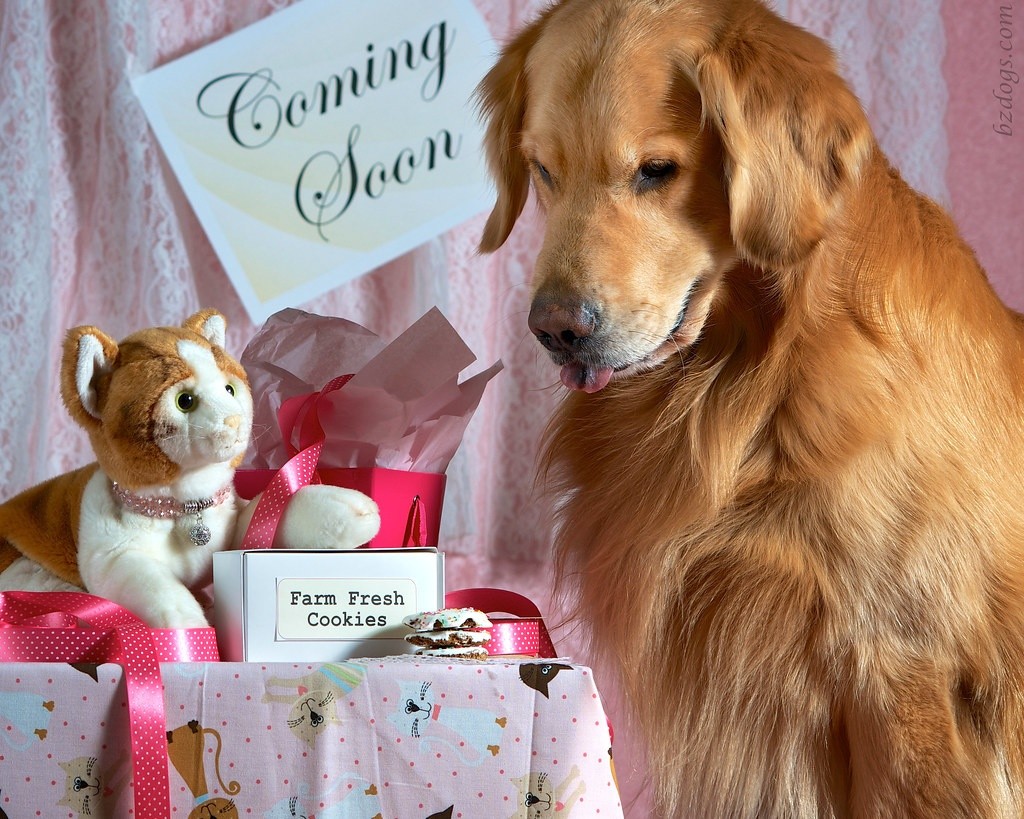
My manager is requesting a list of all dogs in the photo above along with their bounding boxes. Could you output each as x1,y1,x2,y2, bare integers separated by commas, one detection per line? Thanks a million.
464,0,1024,818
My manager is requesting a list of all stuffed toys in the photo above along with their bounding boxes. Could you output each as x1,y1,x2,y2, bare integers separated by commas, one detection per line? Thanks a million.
0,308,383,629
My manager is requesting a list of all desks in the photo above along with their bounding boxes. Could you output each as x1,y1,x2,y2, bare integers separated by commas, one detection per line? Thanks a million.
0,658,624,819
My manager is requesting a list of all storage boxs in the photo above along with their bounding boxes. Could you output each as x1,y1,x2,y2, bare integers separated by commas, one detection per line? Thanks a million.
212,545,444,660
